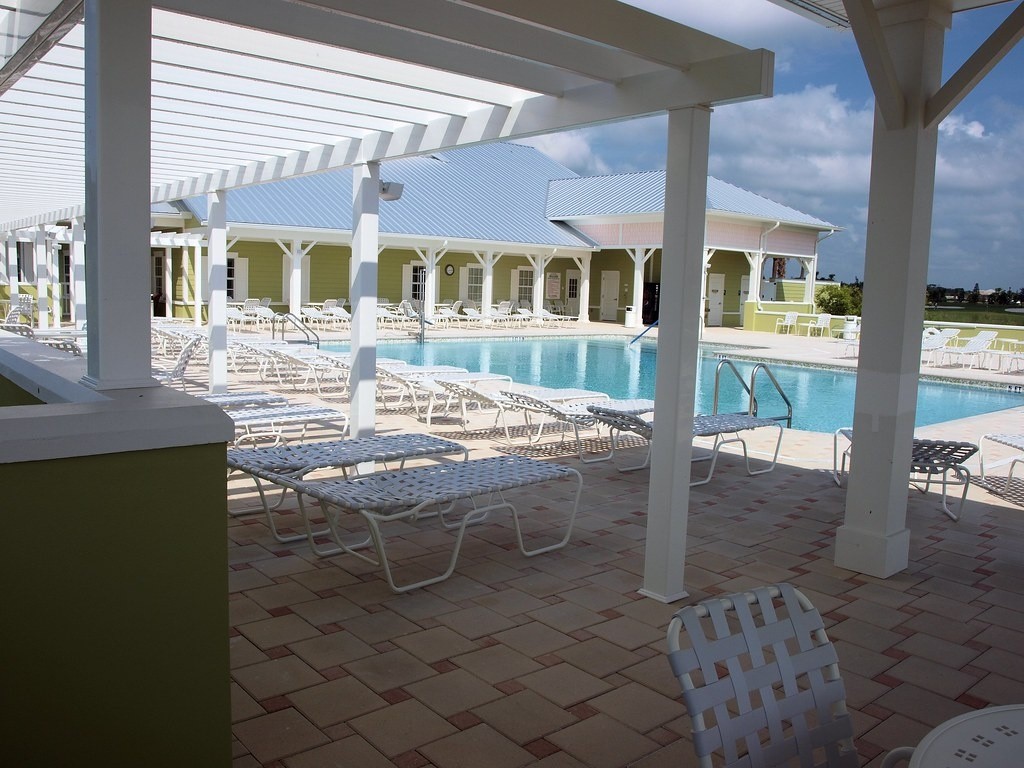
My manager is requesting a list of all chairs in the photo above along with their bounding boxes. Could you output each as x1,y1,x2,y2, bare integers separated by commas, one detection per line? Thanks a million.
799,314,831,337
832,427,1024,523
920,327,1024,376
665,580,861,768
775,311,799,337
828,326,860,360
0,298,794,594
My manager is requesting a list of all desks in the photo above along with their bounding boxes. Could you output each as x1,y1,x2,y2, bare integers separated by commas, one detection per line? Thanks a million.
880,703,1024,768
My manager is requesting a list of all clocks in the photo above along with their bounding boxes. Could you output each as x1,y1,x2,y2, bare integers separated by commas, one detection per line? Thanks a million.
446,265,455,276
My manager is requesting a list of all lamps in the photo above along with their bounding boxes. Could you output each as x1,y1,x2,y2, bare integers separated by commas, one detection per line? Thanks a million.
45,231,56,239
379,179,404,201
